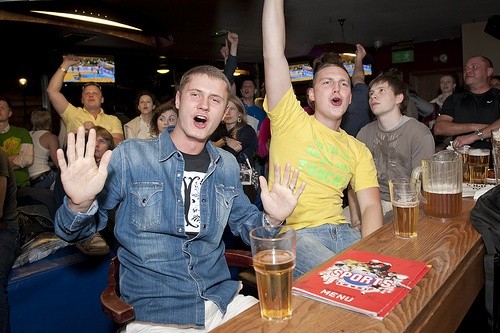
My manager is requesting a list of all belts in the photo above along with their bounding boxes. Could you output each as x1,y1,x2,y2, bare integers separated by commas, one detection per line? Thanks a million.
31,170,50,186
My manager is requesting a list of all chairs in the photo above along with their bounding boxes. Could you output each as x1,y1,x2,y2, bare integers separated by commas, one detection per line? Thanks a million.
100,249,253,324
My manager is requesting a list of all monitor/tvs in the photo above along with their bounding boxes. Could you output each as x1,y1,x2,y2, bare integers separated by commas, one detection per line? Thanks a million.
62,53,117,85
343,63,373,77
288,61,314,83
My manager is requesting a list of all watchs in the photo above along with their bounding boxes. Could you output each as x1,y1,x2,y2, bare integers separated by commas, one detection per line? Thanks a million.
477,128,484,141
223,136,228,146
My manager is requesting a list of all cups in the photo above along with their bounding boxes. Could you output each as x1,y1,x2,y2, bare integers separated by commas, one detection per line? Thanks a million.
410,155,463,220
468,149,490,188
249,227,296,321
491,130,500,185
456,148,470,183
389,178,421,238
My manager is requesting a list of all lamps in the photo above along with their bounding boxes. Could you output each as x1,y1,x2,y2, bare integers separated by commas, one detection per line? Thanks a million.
308,18,357,58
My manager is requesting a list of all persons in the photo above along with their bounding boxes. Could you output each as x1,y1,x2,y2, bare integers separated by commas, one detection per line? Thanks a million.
434,55,500,170
0,30,315,254
54,65,307,333
0,148,22,333
343,72,436,232
422,73,457,151
313,44,374,139
388,68,418,120
263,0,384,280
489,75,500,90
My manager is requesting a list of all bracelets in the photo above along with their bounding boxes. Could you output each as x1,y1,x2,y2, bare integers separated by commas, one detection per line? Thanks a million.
264,212,283,229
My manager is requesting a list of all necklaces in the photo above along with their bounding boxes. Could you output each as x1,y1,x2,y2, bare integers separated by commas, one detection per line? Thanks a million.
142,116,152,127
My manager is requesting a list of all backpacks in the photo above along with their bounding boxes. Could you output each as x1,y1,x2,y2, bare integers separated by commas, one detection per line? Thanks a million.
468,183,499,256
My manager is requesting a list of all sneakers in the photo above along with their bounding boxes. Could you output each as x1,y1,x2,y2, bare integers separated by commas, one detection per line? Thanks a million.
76,232,110,257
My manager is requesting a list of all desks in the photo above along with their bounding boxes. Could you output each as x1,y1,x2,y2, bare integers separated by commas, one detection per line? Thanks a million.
208,177,495,333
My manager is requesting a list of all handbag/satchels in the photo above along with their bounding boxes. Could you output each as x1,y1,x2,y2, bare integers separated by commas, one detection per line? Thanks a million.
239,151,259,191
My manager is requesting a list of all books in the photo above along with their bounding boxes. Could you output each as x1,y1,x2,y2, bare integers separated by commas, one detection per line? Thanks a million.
292,248,433,320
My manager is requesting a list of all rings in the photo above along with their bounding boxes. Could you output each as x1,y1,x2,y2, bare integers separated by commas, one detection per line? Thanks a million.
288,185,296,192
